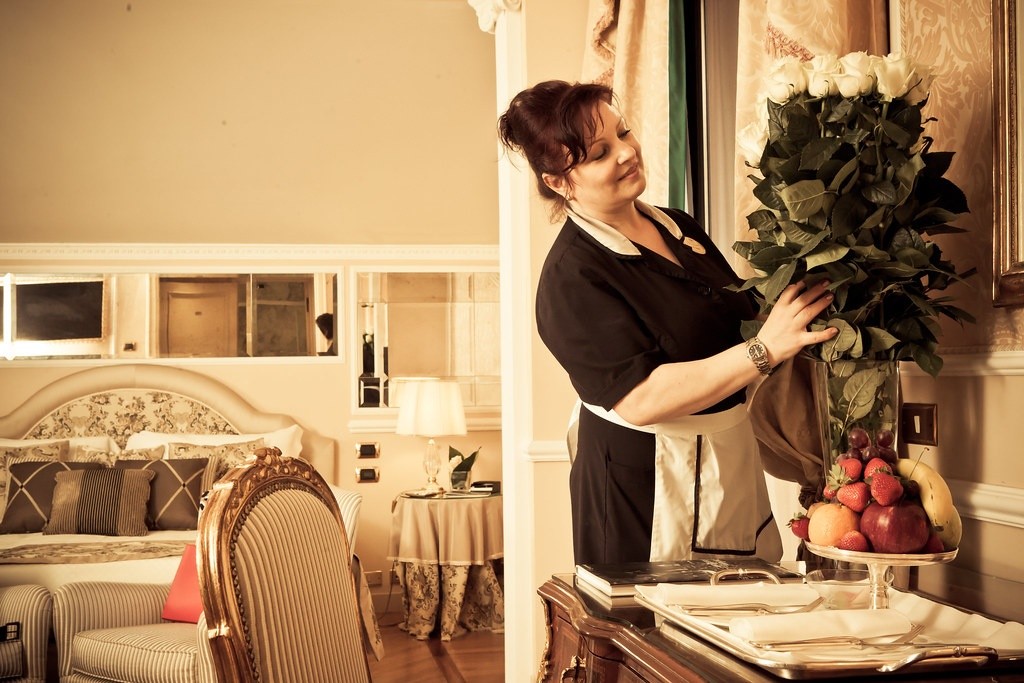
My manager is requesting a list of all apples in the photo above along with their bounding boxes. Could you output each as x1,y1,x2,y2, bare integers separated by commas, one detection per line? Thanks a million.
858,498,930,554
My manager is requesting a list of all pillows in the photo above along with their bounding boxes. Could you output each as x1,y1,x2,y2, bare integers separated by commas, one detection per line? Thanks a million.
125,424,303,460
0,440,69,521
0,456,109,535
161,544,204,623
69,445,165,469
167,438,265,483
41,468,156,536
115,455,218,531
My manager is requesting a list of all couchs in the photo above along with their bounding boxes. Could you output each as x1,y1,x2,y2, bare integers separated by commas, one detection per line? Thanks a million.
51,483,363,683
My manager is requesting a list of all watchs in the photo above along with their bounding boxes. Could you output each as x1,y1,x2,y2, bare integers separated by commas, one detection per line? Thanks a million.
745,336,771,376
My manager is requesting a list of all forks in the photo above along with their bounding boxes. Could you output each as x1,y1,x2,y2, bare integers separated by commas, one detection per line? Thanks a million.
748,625,926,651
677,597,825,615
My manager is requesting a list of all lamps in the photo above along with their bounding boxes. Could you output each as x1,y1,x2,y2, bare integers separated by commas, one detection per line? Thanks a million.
396,381,467,498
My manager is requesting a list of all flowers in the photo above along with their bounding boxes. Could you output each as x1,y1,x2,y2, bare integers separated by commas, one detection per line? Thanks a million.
720,51,980,479
447,446,479,491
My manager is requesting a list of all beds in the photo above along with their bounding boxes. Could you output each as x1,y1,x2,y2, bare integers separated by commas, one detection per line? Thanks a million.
0,364,334,592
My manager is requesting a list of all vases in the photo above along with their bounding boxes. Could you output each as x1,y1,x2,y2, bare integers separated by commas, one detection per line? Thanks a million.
812,359,904,582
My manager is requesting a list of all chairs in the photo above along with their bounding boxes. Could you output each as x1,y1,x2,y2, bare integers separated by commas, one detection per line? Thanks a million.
196,446,373,683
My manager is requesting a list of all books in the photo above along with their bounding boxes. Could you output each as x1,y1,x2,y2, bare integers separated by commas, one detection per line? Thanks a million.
576,558,806,597
575,575,643,609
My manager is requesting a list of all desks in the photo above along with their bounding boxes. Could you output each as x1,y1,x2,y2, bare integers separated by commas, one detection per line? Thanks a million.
536,573,1024,683
386,492,504,641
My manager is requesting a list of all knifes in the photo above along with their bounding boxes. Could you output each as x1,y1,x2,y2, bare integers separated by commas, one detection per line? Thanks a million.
763,643,978,649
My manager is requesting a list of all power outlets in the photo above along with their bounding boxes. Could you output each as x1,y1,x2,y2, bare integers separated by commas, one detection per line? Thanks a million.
364,571,382,585
389,569,400,583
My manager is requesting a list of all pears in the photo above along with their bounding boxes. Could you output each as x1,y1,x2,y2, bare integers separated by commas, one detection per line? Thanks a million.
805,503,858,548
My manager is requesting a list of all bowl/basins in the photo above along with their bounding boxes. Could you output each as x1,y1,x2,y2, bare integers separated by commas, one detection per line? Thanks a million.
805,568,895,611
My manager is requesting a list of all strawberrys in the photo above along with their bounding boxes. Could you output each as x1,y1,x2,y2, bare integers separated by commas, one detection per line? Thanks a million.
824,458,918,512
926,535,944,553
840,530,868,551
786,512,810,541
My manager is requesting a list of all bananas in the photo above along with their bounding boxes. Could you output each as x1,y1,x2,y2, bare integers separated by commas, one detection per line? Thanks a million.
894,459,962,550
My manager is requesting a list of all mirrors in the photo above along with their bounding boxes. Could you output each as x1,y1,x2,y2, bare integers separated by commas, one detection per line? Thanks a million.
0,264,502,430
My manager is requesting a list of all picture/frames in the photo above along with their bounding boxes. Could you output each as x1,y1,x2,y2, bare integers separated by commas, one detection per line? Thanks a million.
989,0,1024,307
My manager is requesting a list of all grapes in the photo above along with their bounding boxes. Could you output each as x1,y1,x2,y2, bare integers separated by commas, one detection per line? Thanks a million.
845,429,897,468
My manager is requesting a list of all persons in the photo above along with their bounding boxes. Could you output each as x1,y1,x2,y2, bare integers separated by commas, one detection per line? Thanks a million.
500,80,839,565
